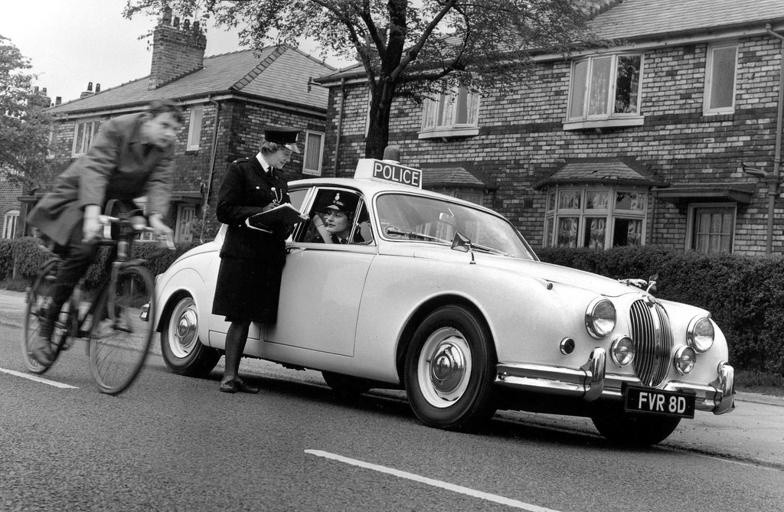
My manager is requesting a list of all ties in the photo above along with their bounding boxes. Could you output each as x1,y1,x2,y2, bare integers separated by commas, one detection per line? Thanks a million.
266,166,271,177
341,239,347,245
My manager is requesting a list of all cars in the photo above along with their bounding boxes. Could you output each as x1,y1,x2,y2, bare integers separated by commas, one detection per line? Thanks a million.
139,155,743,453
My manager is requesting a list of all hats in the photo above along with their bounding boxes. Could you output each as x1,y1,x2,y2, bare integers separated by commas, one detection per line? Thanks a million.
326,192,355,211
264,126,301,154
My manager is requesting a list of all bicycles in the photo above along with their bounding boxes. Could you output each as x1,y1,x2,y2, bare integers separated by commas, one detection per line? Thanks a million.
18,212,178,397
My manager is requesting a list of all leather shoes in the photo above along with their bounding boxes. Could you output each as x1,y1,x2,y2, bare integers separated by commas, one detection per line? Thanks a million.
236,382,260,393
220,379,237,393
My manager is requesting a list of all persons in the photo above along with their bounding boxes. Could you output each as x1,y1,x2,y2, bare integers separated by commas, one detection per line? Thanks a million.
310,191,364,244
212,127,302,394
25,99,185,367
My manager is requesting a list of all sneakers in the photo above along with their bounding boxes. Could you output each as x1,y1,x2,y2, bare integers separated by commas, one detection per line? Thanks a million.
31,335,54,366
